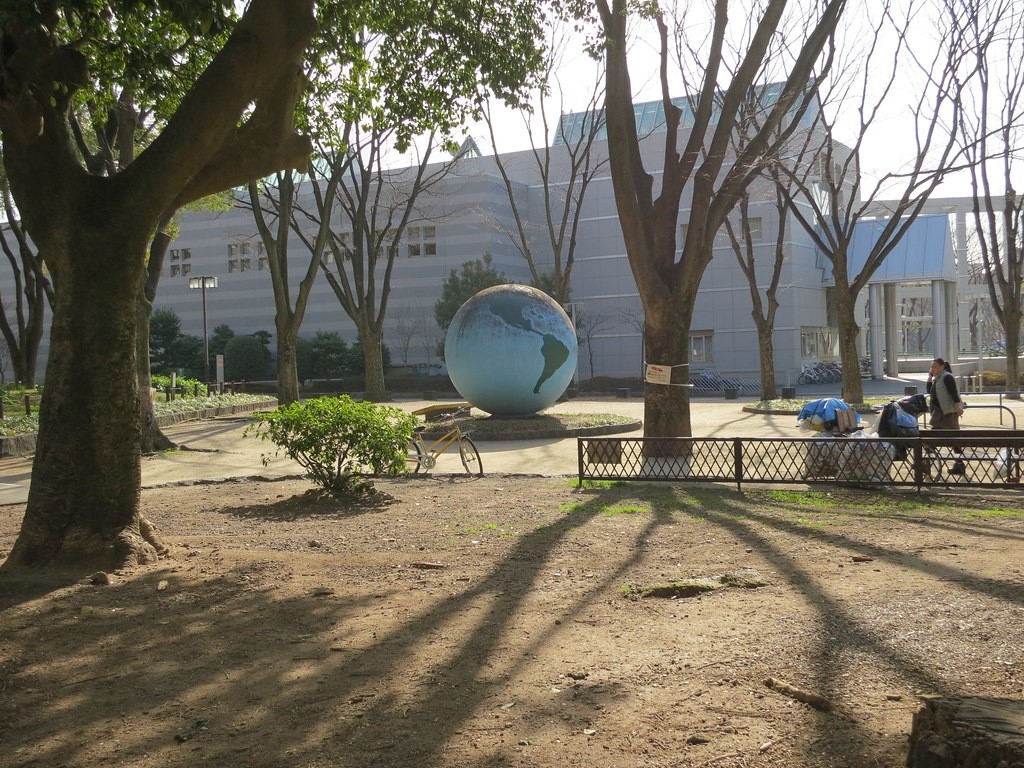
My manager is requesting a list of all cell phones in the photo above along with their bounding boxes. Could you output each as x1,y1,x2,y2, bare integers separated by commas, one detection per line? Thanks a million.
931,373,933,377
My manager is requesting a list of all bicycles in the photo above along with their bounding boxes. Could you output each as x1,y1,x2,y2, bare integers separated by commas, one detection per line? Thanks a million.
798,350,888,385
805,393,942,485
379,408,483,477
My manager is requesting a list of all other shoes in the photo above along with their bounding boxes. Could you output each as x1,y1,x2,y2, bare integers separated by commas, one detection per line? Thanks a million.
948,463,965,474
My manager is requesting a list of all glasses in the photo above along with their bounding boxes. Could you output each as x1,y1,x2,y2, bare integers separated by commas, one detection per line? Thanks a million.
931,364,942,367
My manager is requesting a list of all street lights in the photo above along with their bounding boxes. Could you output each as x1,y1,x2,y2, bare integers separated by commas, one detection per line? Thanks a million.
191,275,219,396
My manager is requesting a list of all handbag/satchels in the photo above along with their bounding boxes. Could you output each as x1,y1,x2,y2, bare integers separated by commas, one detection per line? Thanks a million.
929,410,940,425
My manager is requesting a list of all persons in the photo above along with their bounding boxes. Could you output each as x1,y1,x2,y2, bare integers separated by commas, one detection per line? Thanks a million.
910,357,966,474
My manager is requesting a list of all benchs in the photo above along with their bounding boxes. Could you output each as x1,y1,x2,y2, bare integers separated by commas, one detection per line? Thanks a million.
915,430,1024,491
